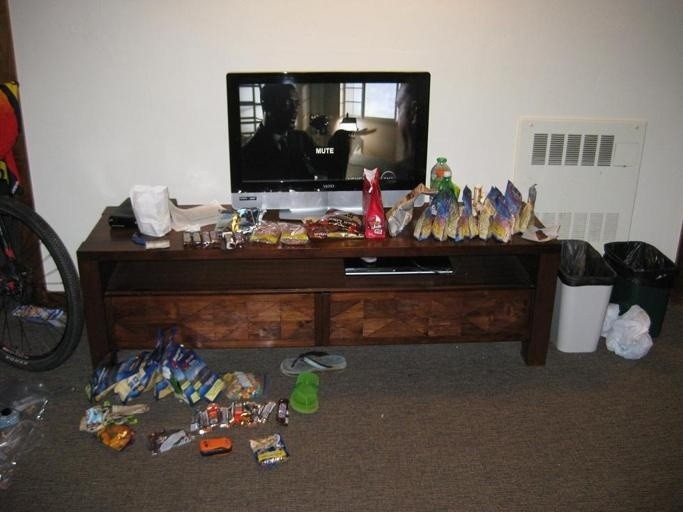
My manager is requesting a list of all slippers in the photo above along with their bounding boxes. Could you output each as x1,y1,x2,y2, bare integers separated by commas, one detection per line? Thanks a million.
280,351,348,375
289,372,320,414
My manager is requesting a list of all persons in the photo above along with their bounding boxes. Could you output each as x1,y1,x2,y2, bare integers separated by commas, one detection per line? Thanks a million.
381,78,424,183
240,82,376,181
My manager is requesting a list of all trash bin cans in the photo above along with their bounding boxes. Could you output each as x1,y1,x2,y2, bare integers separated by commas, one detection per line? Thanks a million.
602,241,679,338
550,239,616,353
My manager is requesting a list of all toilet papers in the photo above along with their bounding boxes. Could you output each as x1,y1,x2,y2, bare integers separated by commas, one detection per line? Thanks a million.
127,184,222,237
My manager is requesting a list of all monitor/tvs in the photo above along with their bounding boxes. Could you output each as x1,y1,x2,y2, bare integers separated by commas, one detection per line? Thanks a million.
225,70,431,193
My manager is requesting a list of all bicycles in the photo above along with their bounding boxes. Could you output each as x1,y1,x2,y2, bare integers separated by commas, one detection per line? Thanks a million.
0,169,84,371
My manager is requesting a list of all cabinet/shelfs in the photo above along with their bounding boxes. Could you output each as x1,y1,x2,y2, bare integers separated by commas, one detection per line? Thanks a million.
78,243,559,367
